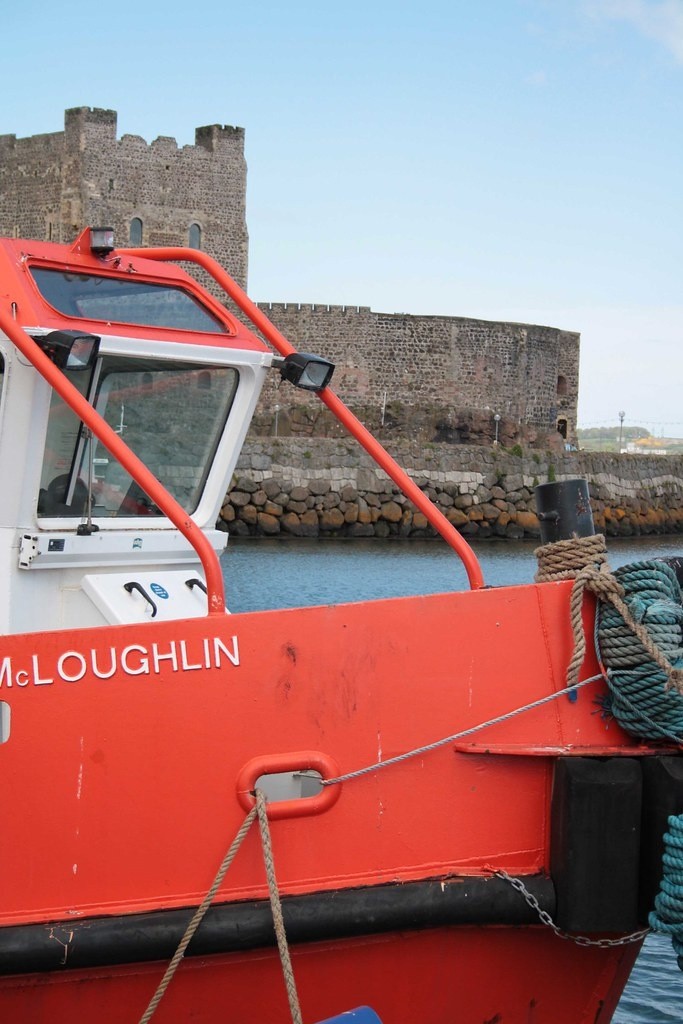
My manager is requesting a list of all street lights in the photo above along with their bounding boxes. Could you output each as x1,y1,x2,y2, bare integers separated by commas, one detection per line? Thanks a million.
619,411,625,453
494,414,501,441
274,405,280,436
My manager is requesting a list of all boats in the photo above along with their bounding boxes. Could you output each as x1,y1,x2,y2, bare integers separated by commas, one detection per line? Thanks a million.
1,227,682,1023
